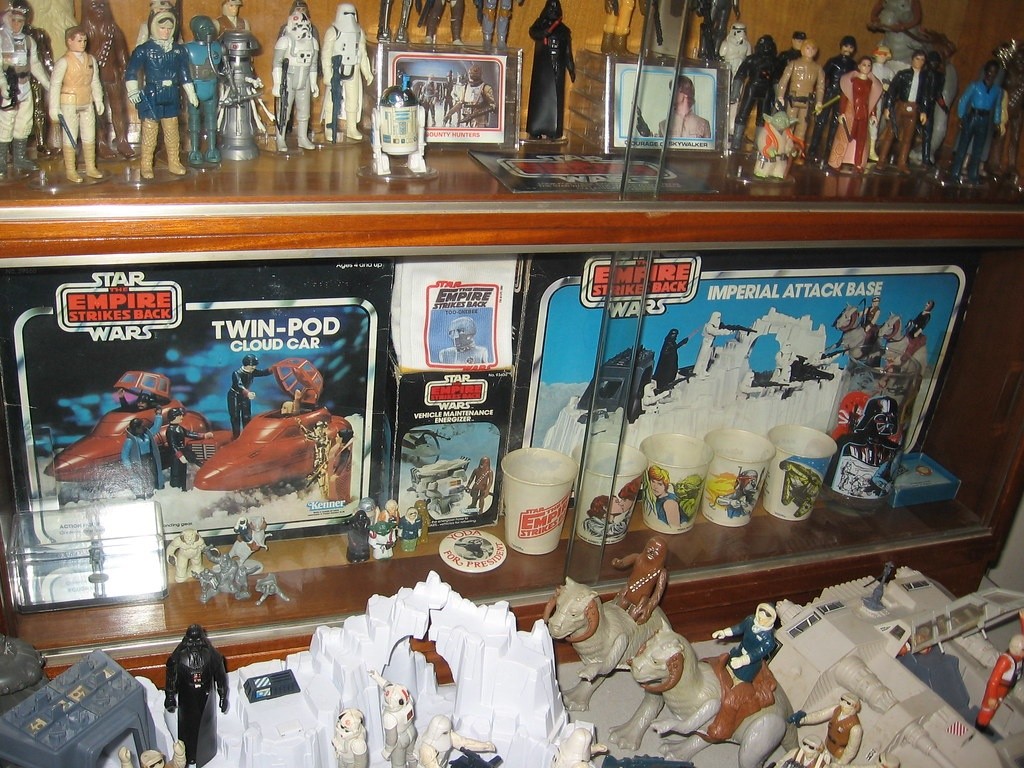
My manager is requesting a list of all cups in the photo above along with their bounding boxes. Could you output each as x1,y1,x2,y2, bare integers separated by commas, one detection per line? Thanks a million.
764,423,838,521
700,429,776,528
819,346,922,518
570,441,648,546
639,433,715,533
501,446,578,555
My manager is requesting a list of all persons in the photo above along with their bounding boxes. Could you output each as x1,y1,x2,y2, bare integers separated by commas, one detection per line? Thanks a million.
612,537,667,625
775,692,901,768
118,740,186,768
659,74,710,138
164,624,229,768
973,634,1024,734
712,602,777,686
552,729,608,768
331,669,496,768
343,497,431,563
0,0,1022,184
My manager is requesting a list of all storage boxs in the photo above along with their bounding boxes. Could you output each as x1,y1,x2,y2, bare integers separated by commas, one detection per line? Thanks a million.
387,347,517,533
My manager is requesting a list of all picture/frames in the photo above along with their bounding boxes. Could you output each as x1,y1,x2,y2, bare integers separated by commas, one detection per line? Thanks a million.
604,56,726,159
376,41,521,154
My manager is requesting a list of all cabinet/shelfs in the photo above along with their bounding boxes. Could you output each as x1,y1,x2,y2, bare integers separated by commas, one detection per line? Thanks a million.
0,139,1024,691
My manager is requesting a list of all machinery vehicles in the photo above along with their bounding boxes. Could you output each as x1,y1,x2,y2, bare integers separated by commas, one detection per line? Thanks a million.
767,558,1024,768
